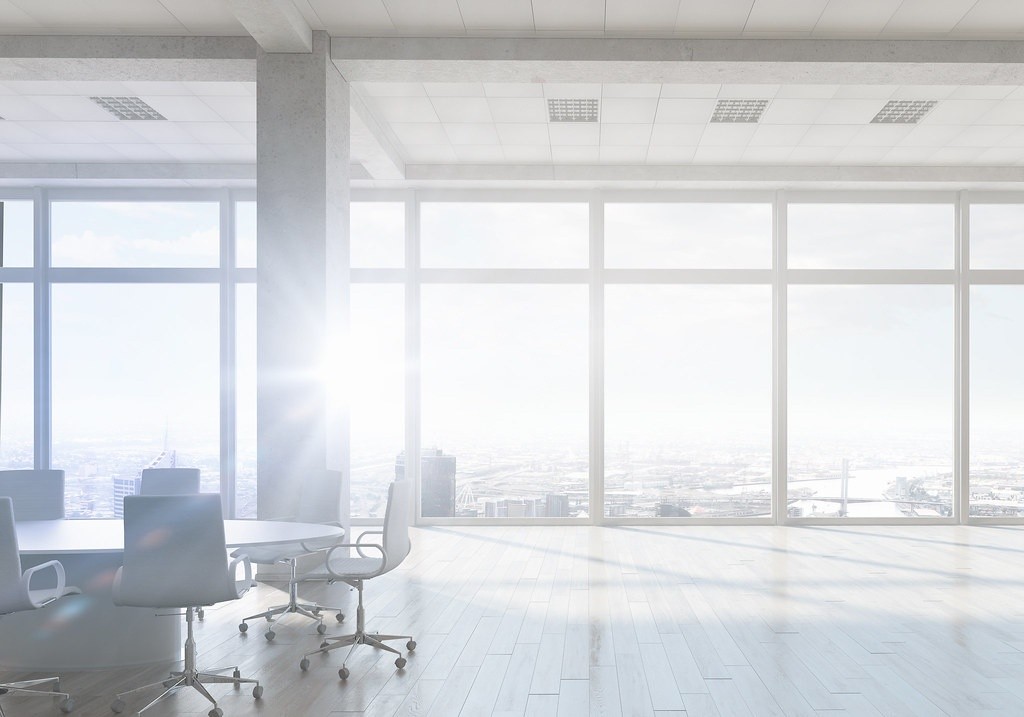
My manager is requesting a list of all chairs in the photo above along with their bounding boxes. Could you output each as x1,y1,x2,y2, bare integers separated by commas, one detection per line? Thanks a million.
138,467,205,621
110,493,263,717
0,496,81,717
300,480,414,679
239,469,344,640
0,470,65,521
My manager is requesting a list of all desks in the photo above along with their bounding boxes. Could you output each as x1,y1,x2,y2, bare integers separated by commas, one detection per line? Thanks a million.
0,519,345,674
